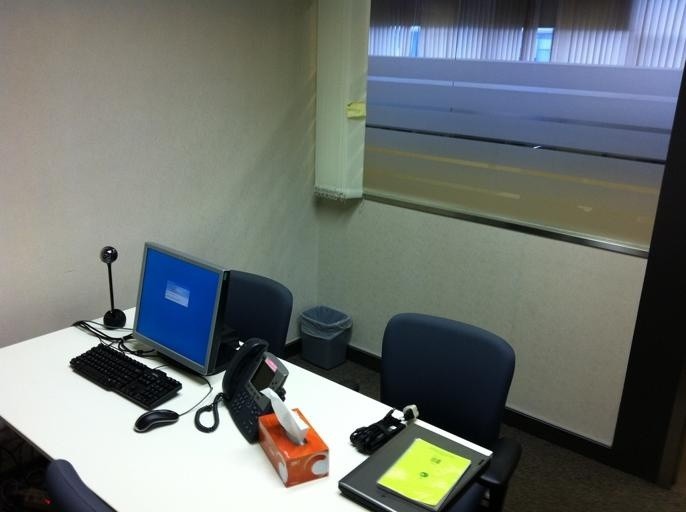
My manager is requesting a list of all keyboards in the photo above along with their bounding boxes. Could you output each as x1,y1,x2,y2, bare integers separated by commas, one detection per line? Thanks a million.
68,342,182,412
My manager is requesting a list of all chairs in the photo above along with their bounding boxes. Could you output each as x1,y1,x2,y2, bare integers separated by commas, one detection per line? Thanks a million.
220,271,291,358
379,314,518,488
42,459,113,511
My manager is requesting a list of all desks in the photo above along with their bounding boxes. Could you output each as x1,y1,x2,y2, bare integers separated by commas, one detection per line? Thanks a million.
0,307,496,512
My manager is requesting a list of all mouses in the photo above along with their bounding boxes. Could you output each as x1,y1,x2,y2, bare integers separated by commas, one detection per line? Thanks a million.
133,409,180,433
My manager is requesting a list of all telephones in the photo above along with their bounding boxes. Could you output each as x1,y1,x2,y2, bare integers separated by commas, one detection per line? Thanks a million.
192,337,290,443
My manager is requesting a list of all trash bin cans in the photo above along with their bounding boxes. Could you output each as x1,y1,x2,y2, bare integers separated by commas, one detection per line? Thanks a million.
299,305,353,370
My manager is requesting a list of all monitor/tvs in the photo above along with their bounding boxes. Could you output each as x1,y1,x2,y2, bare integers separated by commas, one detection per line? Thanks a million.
132,241,237,377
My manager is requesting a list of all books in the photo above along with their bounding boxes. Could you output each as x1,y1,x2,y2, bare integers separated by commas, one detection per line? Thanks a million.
378,437,472,511
